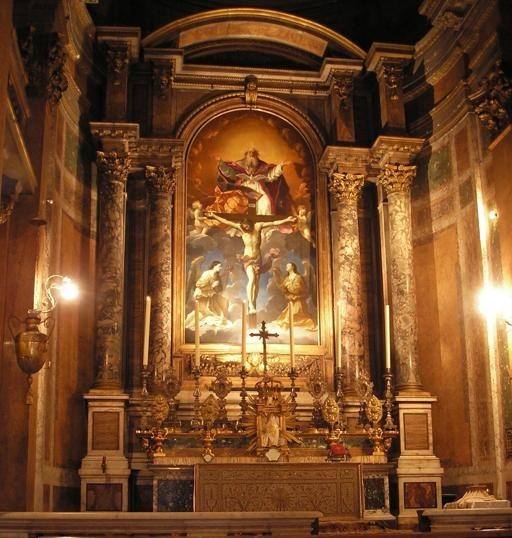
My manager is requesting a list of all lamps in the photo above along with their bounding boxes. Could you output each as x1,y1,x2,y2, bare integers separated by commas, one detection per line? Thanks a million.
38,275,78,317
5,311,59,378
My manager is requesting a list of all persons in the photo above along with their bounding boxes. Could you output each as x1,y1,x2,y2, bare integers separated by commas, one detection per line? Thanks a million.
185,147,317,336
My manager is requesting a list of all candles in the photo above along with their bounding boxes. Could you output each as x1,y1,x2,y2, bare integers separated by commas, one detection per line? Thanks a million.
289,301,294,367
195,300,201,368
336,302,342,369
385,305,391,369
143,295,151,369
242,300,246,367
